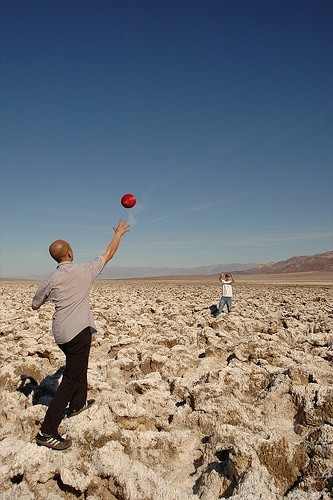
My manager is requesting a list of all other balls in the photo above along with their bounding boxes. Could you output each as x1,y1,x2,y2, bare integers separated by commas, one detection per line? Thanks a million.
120,193,137,209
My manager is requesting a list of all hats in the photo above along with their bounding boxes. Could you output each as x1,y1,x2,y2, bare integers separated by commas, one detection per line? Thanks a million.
225,273,230,277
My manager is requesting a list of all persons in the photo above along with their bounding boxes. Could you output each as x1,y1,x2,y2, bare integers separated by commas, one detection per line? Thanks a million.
217,272,234,315
32,219,132,449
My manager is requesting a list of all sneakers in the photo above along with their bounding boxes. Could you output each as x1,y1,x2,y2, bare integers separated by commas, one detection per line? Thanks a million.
35,430,72,449
71,399,95,416
